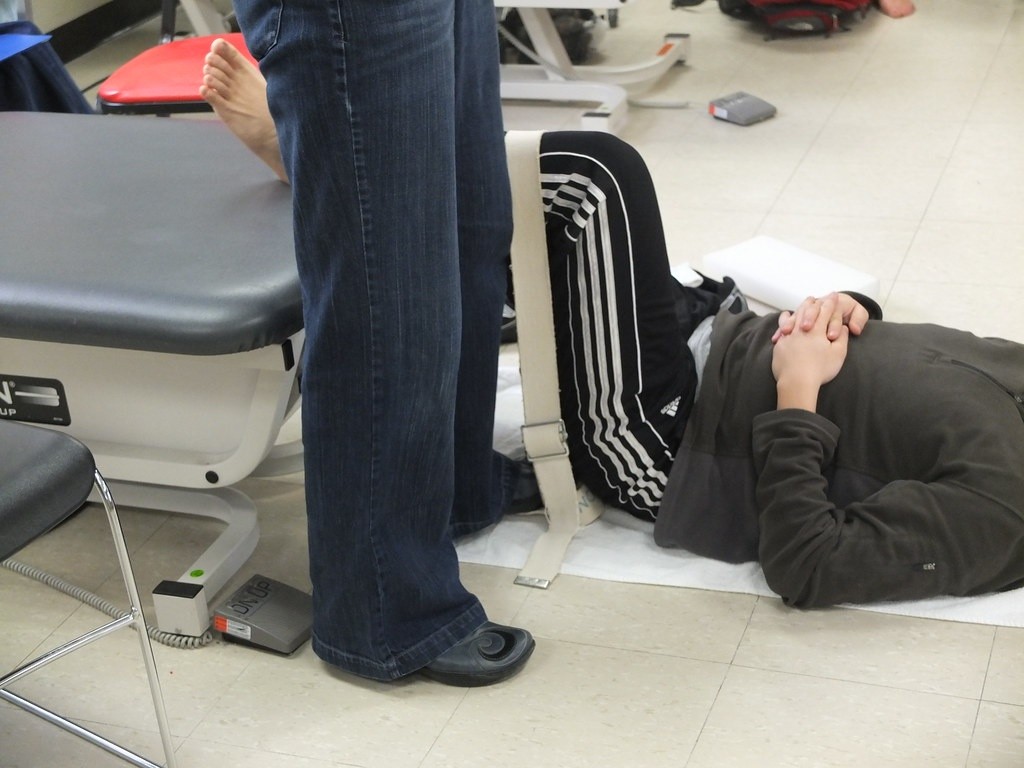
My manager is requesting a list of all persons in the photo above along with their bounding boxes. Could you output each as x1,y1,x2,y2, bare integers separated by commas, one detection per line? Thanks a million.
233,1,550,688
199,39,1024,611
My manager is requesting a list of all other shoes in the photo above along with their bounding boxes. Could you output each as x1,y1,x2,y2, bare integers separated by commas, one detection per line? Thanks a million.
417,620,535,688
506,460,583,514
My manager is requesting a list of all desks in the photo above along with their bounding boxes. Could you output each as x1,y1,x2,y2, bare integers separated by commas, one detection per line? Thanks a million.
0,110,304,489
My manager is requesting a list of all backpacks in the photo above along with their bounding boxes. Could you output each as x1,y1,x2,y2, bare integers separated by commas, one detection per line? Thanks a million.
719,0,873,42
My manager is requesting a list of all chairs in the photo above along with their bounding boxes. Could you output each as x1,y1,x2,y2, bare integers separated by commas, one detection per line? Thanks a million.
0,420,176,768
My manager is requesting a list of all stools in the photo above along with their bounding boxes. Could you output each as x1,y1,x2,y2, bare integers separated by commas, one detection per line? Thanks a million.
97,32,258,118
